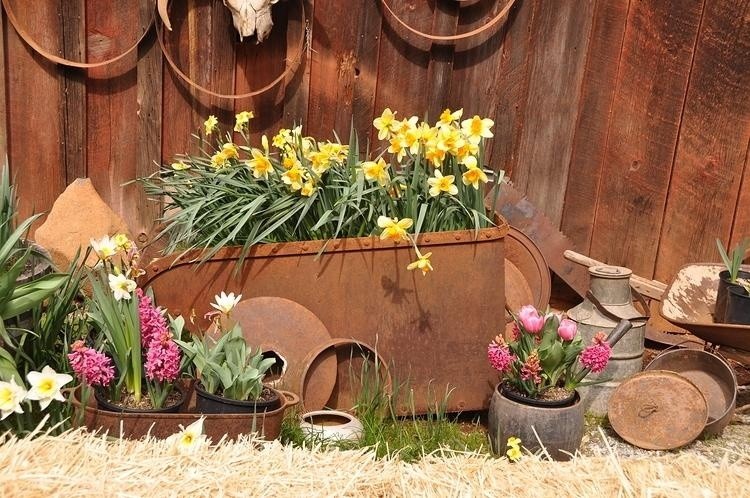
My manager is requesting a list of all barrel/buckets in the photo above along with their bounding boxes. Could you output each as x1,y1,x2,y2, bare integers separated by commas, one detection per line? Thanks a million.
569,267,650,424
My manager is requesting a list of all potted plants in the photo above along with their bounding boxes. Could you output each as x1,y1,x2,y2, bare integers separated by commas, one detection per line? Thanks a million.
711,236,750,325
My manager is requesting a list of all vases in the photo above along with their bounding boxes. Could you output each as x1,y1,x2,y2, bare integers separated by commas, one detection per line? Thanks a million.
300,408,365,452
489,377,585,461
724,285,750,325
92,386,184,414
191,381,280,415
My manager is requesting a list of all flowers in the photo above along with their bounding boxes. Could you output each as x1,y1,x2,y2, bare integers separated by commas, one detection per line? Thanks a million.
69,233,197,404
487,302,613,396
120,104,511,280
177,286,277,398
